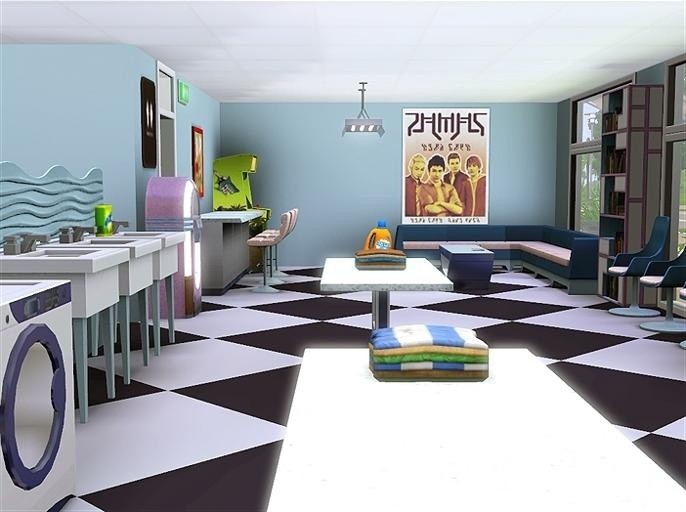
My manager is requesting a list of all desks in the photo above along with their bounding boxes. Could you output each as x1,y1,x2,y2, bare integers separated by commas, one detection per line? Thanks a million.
318,257,454,337
259,345,686,511
195,208,266,298
437,239,496,292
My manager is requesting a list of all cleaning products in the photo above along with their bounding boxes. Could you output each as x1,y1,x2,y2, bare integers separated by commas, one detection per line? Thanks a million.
363,220,394,250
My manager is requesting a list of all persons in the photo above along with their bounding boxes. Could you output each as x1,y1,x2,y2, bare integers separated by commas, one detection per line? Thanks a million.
406,153,486,217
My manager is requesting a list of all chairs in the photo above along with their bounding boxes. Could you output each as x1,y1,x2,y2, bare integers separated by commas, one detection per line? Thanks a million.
245,204,300,295
636,242,686,337
678,280,686,350
607,214,673,319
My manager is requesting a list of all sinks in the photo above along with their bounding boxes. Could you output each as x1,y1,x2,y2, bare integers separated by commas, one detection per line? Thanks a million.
118,232,161,236
21,249,100,257
75,239,136,244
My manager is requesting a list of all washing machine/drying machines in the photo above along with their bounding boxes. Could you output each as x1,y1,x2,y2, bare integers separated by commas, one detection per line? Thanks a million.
0,279,76,512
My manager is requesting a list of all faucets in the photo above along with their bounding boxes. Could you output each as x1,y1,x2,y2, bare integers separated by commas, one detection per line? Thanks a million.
20,232,51,254
73,226,98,242
112,219,129,234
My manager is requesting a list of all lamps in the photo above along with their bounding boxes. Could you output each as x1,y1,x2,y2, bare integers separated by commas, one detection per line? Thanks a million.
338,79,388,141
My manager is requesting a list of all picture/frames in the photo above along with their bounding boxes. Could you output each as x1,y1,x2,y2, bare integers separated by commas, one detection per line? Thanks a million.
190,123,206,200
138,75,159,169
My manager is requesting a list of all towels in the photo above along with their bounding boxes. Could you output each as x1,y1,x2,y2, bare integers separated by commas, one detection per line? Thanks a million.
367,321,490,385
354,247,406,272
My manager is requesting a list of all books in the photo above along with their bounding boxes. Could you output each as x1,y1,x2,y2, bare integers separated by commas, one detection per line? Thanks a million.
603,110,628,302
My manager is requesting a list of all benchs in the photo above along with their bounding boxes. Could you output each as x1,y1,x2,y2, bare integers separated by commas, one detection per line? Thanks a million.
522,223,617,296
391,222,545,272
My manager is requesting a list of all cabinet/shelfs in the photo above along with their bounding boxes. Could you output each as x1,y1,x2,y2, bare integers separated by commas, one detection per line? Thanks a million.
594,82,666,307
